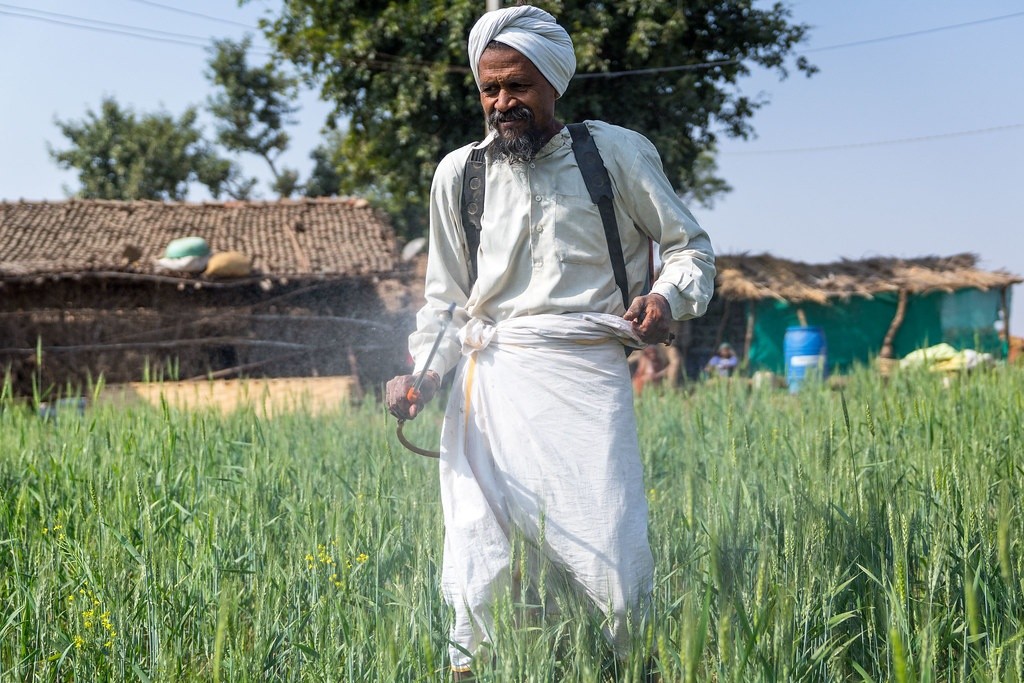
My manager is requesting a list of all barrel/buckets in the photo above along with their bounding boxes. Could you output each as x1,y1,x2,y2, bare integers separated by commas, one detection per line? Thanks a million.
783,326,826,394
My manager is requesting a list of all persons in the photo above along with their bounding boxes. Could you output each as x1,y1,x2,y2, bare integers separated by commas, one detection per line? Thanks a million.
705,342,737,378
627,342,681,396
386,5,717,683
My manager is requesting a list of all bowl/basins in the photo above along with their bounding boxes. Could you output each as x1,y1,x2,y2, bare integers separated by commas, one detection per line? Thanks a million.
165,237,209,256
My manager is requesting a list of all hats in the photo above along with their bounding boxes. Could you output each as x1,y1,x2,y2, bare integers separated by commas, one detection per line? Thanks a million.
468,5,576,100
